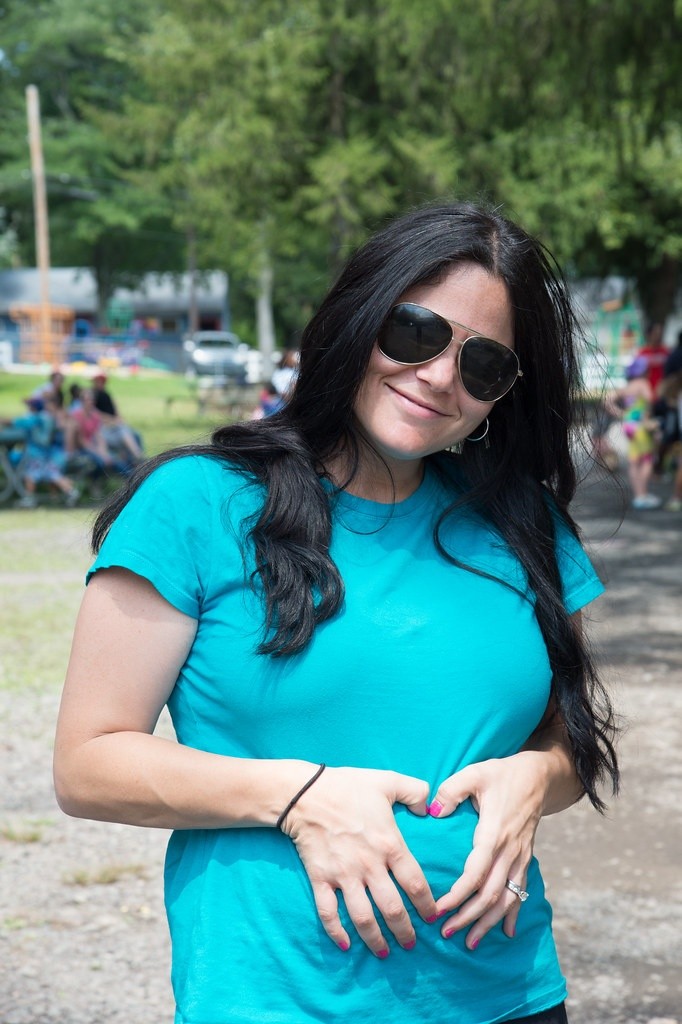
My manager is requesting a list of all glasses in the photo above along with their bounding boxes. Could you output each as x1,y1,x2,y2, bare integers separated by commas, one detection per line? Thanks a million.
377,303,520,404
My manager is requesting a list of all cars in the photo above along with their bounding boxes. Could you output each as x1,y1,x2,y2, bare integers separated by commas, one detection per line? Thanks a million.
183,331,251,376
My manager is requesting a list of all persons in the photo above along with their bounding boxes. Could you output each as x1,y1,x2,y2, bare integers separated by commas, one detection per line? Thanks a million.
234,346,302,421
1,370,148,509
600,320,682,515
52,206,629,1024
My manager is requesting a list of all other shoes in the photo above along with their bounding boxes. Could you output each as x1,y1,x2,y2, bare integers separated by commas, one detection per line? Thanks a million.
632,493,659,507
66,488,83,505
18,497,38,508
663,499,682,511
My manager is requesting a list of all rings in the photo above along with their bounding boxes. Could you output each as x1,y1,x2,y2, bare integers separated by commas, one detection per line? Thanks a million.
505,878,529,902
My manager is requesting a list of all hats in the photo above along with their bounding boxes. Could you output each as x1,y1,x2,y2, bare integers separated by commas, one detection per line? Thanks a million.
24,396,43,410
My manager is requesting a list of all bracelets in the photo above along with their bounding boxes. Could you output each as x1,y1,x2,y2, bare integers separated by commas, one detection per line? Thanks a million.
276,762,326,831
